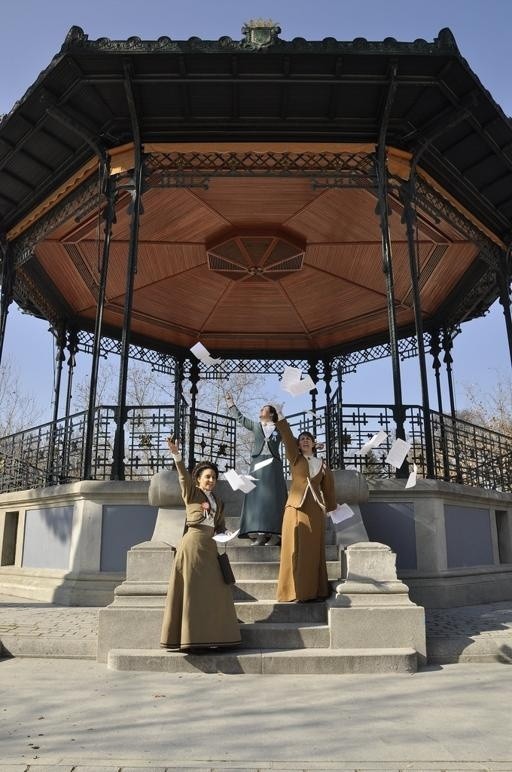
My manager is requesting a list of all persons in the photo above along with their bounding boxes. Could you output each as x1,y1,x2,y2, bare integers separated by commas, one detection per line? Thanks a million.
226,392,288,546
274,401,337,602
160,437,242,651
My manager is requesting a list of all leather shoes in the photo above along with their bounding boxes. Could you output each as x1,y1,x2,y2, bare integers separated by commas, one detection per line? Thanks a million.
251,538,267,546
265,537,280,546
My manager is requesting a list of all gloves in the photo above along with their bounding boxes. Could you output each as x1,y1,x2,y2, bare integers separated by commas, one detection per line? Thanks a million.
274,402,285,421
225,391,234,408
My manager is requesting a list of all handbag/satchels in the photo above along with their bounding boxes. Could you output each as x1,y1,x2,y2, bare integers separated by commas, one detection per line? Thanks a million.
217,553,235,584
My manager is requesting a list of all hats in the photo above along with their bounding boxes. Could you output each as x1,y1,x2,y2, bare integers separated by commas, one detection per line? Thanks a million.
192,461,218,480
